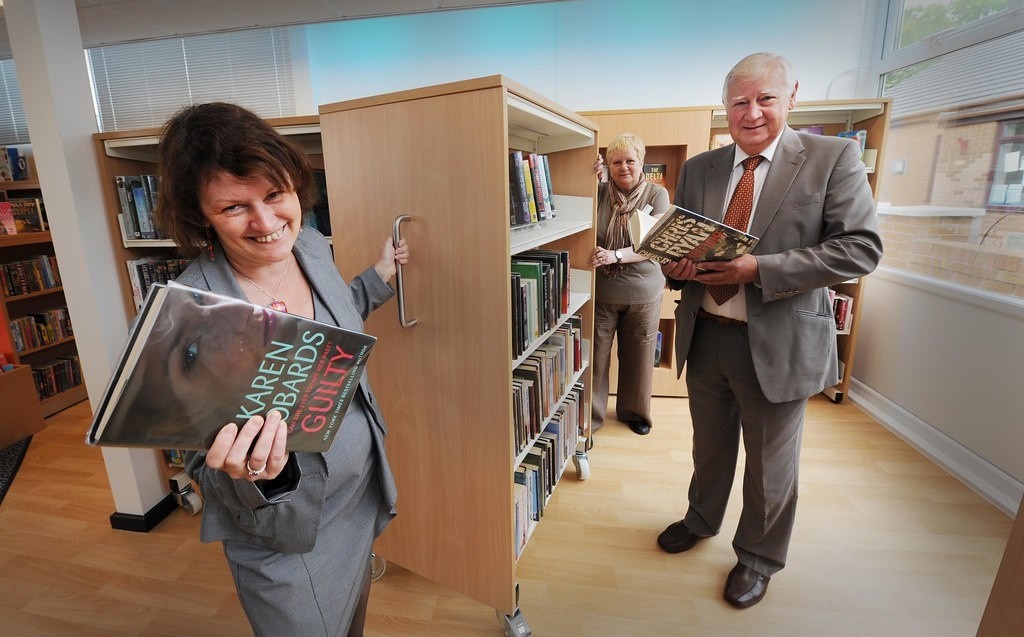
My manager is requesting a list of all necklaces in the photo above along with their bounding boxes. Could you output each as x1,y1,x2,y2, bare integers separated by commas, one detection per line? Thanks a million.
242,255,290,313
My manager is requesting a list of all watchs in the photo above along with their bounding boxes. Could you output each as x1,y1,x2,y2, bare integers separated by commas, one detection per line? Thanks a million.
614,249,622,264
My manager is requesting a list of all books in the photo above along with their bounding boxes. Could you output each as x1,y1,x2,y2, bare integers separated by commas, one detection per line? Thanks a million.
829,288,853,332
627,206,759,276
643,164,667,187
115,173,195,465
301,168,331,236
509,151,585,562
601,166,608,183
0,146,84,399
711,133,734,151
86,282,376,453
794,124,868,163
654,331,663,367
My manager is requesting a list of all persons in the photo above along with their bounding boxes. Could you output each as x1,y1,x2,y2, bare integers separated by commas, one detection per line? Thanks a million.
151,101,409,637
657,51,884,608
124,291,369,452
590,135,670,436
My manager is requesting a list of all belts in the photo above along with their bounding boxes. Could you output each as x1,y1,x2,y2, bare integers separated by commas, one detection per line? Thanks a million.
697,311,748,326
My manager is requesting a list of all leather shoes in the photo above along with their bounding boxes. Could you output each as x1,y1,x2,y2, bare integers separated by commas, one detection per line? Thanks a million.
724,560,771,609
630,420,649,435
657,520,699,553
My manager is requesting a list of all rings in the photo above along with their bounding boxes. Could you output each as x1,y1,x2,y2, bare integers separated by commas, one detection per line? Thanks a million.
597,257,603,263
247,460,266,476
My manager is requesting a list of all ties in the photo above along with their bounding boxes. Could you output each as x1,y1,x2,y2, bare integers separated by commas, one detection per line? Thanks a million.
704,156,763,307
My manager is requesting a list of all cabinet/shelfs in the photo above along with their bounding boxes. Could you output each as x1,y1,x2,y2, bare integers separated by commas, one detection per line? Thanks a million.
91,112,327,517
318,76,597,636
574,104,712,397
706,98,890,405
0,176,91,418
